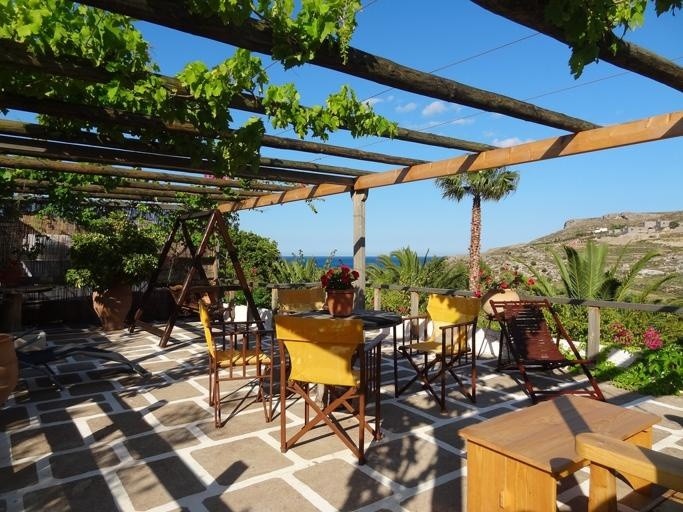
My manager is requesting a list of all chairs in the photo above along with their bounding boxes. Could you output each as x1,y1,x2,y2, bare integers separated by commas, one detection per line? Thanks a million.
575,431,683,512
398,294,479,417
198,299,274,426
13,326,152,390
274,289,326,314
276,313,391,458
489,299,606,405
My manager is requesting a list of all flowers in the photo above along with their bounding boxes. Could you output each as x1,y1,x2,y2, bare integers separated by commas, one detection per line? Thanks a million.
475,265,534,297
5,257,20,269
320,266,359,289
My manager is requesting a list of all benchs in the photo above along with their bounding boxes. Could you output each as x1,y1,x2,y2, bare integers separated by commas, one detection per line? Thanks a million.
166,284,232,323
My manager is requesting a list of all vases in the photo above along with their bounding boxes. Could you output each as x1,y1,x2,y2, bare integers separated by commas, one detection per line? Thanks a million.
481,289,520,317
2,269,21,287
0,334,20,402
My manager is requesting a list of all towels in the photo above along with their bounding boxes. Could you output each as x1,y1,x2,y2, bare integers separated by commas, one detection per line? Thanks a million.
15,332,47,351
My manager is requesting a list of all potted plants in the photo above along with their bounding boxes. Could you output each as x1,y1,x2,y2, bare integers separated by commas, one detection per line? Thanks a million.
66,219,159,331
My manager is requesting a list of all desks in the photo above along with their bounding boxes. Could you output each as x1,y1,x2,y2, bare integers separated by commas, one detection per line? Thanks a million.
477,314,511,362
22,285,54,326
270,311,403,415
458,395,662,512
